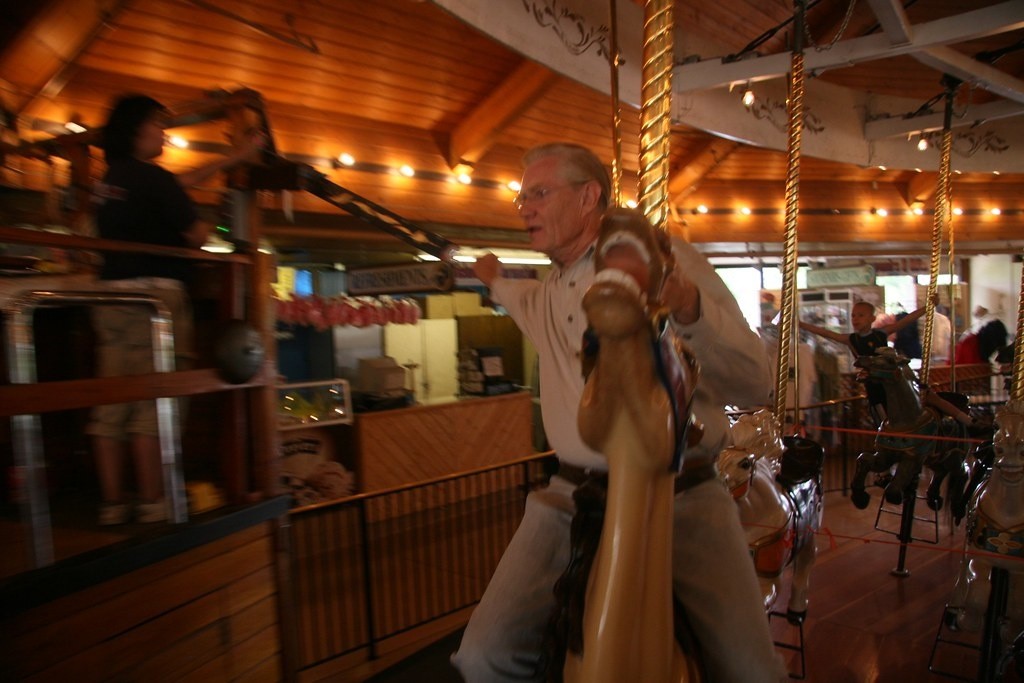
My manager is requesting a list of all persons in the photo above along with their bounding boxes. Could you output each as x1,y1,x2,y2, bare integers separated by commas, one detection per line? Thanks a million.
454,140,797,683
75,95,209,522
793,295,1015,491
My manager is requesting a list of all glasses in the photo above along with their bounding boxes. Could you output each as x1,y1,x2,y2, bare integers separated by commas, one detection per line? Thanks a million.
513,179,609,212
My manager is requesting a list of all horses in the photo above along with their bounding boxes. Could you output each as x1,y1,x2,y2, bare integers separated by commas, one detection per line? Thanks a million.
713,409,825,626
552,207,705,683
939,401,1024,631
846,347,976,518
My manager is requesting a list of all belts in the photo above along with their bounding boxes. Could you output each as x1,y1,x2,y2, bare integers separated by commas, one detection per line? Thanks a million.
550,461,718,495
